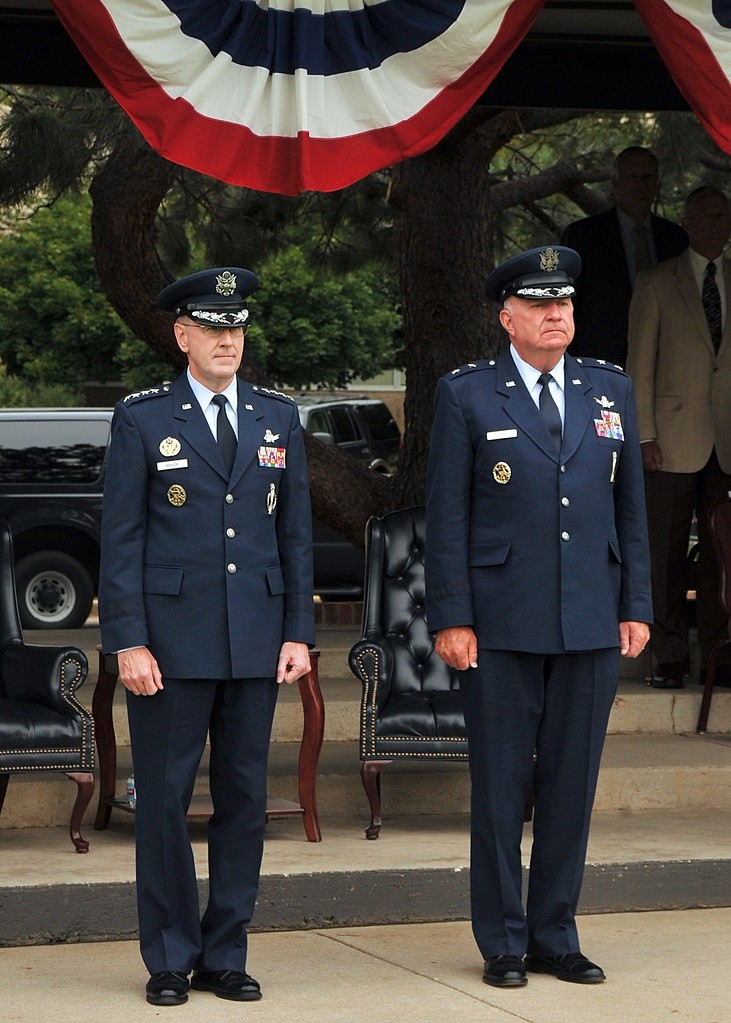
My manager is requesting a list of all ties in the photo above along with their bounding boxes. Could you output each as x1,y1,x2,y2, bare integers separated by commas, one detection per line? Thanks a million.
702,261,722,356
212,395,237,478
536,374,562,453
632,224,652,271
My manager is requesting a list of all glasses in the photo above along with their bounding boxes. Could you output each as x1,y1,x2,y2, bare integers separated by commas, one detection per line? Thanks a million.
177,322,250,338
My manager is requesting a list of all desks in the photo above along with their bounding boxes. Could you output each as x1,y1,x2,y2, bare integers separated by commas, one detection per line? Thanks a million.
92,644,325,844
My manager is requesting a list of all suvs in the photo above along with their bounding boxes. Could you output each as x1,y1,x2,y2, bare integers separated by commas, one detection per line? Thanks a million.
0,406,116,629
297,396,401,472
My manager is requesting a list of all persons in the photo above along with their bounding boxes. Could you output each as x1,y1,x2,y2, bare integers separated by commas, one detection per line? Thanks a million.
97,265,317,1006
561,147,694,624
623,184,731,691
421,244,656,991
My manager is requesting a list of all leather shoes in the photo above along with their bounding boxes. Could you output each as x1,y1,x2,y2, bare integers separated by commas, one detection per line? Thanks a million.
191,969,262,1001
145,971,189,1006
700,664,731,688
482,955,528,987
651,663,682,689
524,949,606,984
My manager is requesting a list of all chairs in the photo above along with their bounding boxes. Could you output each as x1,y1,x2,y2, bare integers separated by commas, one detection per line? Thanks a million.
347,505,538,841
697,489,731,735
0,519,96,854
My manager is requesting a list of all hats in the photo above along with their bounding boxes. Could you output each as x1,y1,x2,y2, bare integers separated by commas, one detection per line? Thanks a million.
484,247,582,307
155,267,258,327
